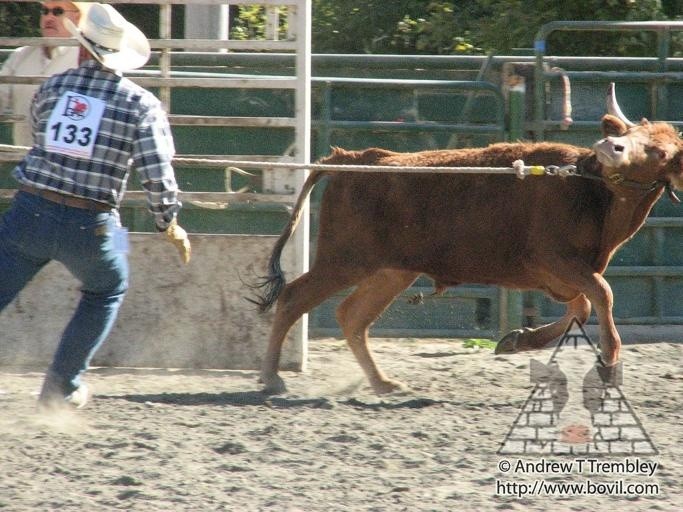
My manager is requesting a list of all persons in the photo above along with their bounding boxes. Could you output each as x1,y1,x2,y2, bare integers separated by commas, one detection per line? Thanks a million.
0,0,99,167
0,1,191,409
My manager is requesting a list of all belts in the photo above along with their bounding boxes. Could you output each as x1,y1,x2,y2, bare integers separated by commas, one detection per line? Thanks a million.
18,179,118,215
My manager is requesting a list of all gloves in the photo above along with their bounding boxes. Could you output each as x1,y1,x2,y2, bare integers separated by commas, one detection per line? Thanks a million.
163,222,192,266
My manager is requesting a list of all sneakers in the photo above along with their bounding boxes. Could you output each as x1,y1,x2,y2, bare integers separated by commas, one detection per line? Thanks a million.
35,385,89,414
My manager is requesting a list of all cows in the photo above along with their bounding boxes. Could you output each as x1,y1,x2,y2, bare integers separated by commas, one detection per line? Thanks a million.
237,82,683,394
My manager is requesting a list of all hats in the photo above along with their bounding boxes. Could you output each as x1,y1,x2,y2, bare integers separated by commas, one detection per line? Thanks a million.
61,3,151,71
38,0,97,12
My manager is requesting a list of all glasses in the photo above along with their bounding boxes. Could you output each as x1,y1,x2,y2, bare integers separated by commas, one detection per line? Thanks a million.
37,7,79,16
